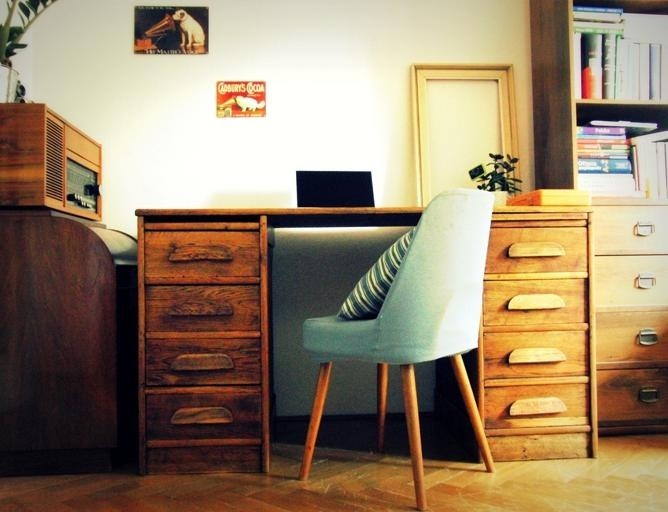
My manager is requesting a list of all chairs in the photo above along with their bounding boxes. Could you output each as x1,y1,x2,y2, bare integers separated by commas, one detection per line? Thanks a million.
300,191,495,511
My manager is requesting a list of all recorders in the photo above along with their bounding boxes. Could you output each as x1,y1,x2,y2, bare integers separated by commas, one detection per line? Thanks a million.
1,103,103,222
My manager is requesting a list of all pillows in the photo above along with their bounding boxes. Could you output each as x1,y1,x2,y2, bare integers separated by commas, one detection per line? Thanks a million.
333,228,414,319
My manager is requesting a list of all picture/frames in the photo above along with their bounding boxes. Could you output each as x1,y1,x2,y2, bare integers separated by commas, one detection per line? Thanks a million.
409,61,523,209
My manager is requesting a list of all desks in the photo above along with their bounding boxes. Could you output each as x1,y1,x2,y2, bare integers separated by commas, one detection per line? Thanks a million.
134,206,595,471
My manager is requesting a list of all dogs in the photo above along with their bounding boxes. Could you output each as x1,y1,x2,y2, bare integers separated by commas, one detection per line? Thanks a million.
172,8,204,49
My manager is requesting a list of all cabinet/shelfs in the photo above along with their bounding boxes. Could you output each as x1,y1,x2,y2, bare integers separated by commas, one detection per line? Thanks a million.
593,202,668,435
528,0,667,192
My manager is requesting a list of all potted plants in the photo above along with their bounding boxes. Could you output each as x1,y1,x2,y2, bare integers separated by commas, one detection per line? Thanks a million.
1,0,55,104
469,153,523,205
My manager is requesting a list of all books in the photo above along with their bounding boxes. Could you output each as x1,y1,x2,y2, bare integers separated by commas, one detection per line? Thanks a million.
573,3,668,103
576,118,668,202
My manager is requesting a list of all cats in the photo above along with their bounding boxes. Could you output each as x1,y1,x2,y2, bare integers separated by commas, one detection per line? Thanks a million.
236,95,266,112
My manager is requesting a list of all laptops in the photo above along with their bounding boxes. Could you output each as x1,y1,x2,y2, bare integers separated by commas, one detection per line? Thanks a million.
296,171,375,208
